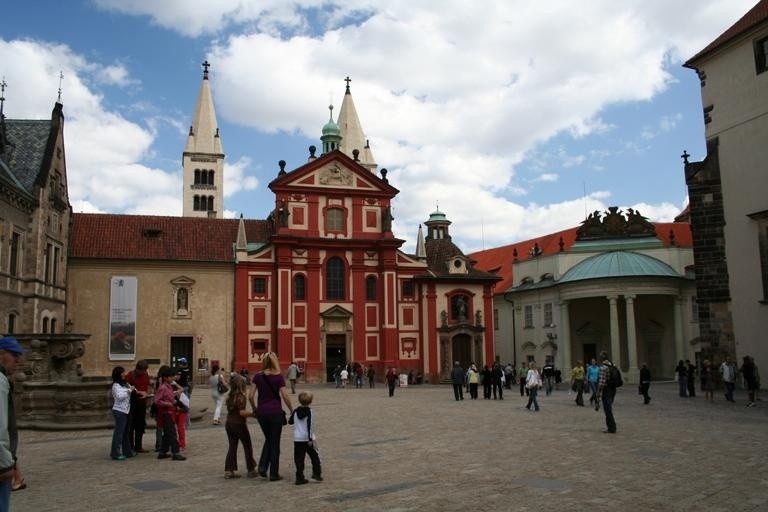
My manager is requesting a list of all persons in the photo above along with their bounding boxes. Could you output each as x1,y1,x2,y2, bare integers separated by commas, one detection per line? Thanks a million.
516,362,554,411
701,360,716,400
385,368,395,397
720,358,738,402
452,361,466,401
740,356,759,407
178,290,186,309
278,203,290,227
11,456,26,491
335,362,375,389
457,296,466,316
467,362,514,401
383,208,394,231
112,358,191,460
602,362,624,433
210,353,322,484
571,358,609,411
640,364,653,403
674,360,697,397
0,336,29,512
408,369,422,384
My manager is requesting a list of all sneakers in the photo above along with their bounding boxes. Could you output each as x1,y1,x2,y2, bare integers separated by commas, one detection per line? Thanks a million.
258,472,268,479
271,476,283,481
158,453,171,459
113,448,149,460
296,480,309,485
172,455,186,461
225,470,241,479
248,471,258,478
311,474,325,483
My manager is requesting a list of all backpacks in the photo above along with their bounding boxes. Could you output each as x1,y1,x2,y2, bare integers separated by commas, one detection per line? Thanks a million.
603,361,623,388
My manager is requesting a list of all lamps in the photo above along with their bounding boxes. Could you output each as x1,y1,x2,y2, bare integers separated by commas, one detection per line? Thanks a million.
64,318,73,334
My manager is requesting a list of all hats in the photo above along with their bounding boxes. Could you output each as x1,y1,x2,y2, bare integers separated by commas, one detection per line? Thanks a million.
0,336,27,353
177,358,187,362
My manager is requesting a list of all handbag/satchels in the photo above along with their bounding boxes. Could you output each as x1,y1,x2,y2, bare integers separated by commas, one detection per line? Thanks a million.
177,392,189,411
526,375,538,389
217,381,228,393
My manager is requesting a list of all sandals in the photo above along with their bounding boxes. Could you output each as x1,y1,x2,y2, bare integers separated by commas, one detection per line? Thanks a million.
11,479,26,492
